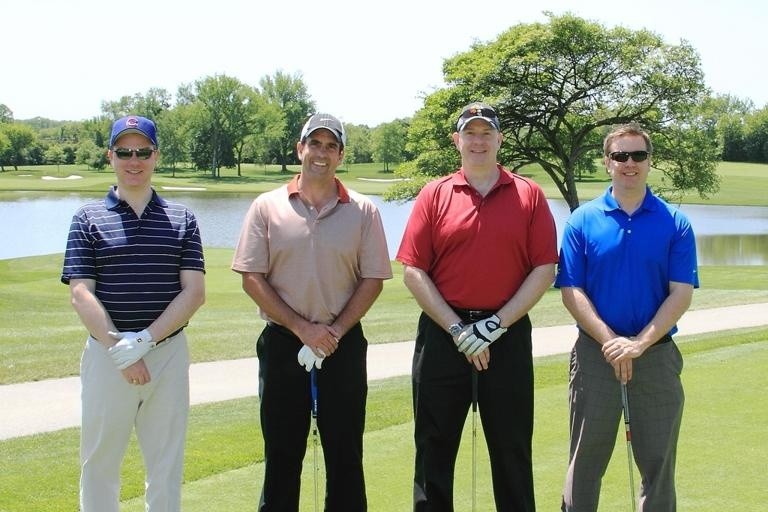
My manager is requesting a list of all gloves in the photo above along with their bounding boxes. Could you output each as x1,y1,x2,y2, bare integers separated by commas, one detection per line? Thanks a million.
297,337,339,372
106,329,156,370
456,314,508,357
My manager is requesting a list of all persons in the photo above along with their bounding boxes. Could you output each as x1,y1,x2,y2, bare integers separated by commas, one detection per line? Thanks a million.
60,115,206,512
554,125,699,511
395,101,560,512
231,111,393,512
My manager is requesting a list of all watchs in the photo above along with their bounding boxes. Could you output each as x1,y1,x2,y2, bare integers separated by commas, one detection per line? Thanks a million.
448,319,466,337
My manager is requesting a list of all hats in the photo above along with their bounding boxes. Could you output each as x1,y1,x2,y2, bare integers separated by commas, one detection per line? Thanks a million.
456,101,500,132
110,115,157,146
300,113,346,147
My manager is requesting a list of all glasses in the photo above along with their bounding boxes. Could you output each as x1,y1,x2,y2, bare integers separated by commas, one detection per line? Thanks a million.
609,150,648,161
114,148,157,160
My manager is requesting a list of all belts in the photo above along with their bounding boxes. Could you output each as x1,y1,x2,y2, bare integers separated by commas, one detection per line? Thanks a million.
456,309,497,320
